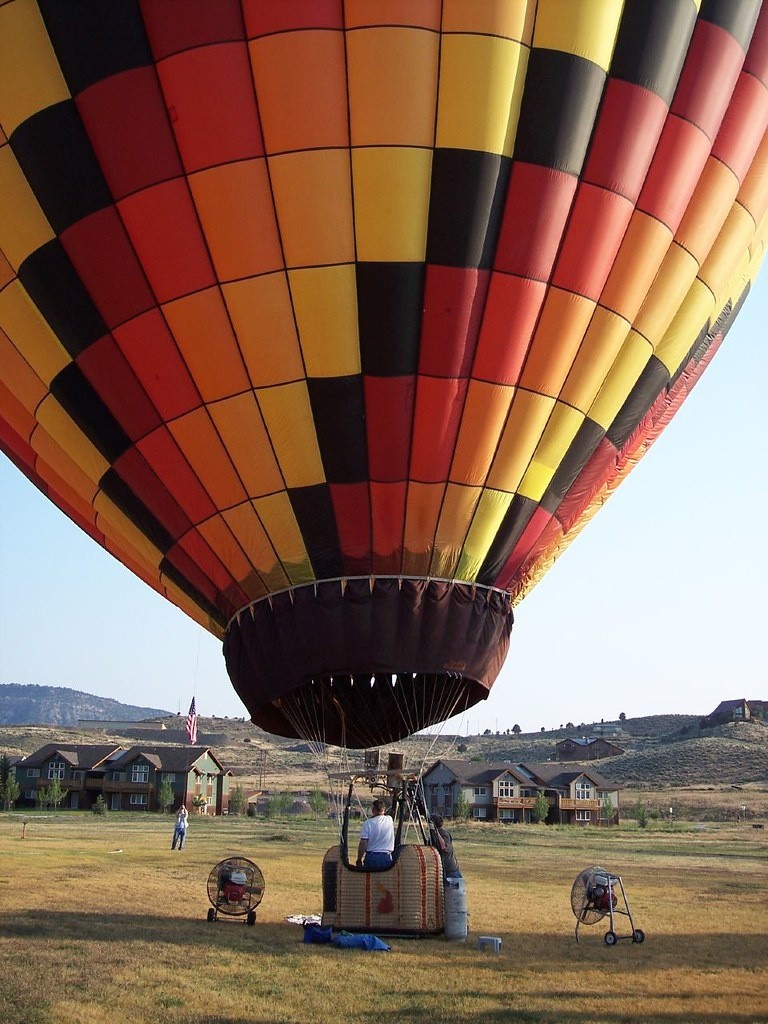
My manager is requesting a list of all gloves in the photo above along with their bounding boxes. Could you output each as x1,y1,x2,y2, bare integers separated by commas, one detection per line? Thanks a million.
429,821,436,830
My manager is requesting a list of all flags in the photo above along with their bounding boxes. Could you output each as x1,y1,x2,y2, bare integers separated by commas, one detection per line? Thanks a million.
186,697,197,745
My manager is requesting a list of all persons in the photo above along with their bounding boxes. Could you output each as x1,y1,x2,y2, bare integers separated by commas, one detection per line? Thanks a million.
171,805,189,850
356,801,395,867
426,814,469,930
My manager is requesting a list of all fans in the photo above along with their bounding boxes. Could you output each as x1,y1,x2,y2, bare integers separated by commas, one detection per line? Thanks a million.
206,857,266,926
571,866,644,945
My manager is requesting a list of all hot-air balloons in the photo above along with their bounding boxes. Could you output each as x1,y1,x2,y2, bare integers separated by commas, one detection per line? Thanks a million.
0,0,768,938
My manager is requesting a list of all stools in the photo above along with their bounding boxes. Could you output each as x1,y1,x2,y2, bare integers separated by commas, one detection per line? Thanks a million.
478,936,502,953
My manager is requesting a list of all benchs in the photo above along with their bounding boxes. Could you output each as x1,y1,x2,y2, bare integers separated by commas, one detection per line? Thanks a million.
753,825,764,831
